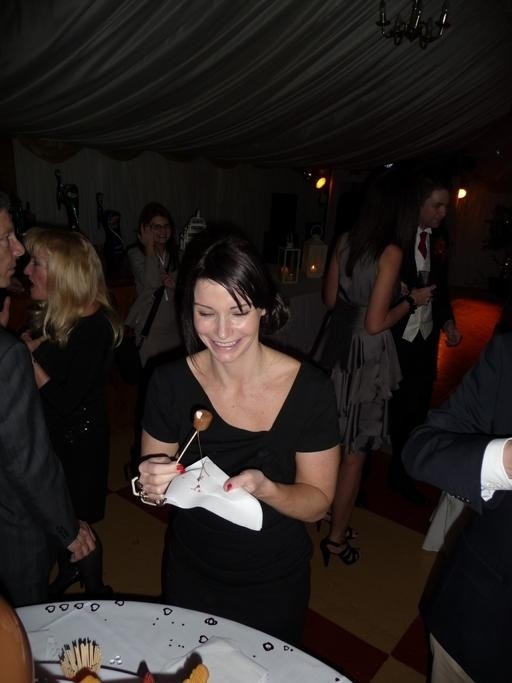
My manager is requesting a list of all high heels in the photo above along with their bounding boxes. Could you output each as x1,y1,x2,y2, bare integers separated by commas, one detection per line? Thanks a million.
317,512,358,540
319,537,360,567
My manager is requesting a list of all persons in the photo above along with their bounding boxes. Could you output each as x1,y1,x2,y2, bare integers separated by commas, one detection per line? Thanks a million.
0,171,511,683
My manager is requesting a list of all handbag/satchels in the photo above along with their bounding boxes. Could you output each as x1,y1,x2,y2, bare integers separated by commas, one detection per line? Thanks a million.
113,324,139,383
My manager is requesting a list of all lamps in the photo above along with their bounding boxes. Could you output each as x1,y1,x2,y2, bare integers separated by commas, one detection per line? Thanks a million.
377,0,451,50
279,224,328,285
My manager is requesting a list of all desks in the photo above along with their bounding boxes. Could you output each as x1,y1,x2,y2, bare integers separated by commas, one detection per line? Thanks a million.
279,280,323,354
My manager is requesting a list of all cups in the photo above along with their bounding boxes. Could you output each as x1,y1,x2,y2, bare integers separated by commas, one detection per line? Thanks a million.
30,310,48,369
415,270,433,288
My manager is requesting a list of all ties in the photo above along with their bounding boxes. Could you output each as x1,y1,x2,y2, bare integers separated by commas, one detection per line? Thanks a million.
418,231,427,259
